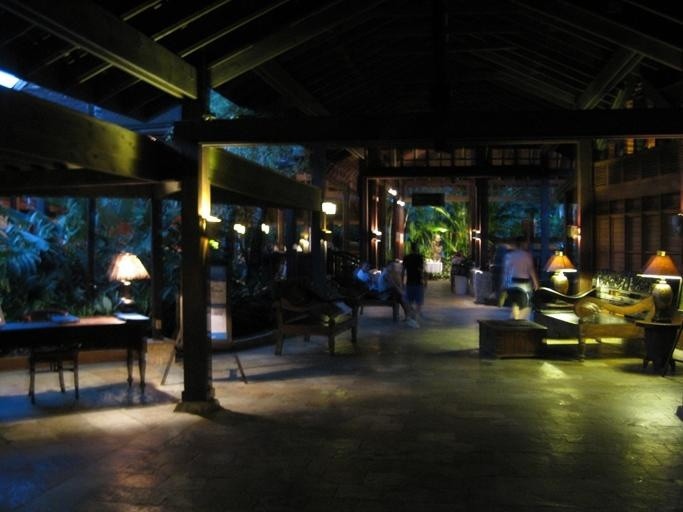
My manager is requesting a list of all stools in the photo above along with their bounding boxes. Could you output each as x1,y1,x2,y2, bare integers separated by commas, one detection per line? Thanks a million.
478,317,548,357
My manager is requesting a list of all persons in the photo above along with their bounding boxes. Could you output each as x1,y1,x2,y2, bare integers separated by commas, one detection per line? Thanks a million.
378,260,419,329
452,252,465,267
402,242,428,321
355,260,380,291
499,236,538,320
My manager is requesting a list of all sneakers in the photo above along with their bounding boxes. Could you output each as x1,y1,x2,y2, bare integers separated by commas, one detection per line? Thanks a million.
407,319,420,330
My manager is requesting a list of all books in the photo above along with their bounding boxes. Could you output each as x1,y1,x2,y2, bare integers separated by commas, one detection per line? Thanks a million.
113,312,150,321
51,314,80,324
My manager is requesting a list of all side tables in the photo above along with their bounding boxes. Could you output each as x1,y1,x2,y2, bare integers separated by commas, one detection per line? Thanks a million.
626,311,683,373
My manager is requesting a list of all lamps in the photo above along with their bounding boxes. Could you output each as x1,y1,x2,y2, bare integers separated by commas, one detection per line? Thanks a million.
541,250,579,295
109,250,153,314
638,251,683,322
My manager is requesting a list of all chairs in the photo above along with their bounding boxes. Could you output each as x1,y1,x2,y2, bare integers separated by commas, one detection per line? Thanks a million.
24,308,83,402
276,278,359,358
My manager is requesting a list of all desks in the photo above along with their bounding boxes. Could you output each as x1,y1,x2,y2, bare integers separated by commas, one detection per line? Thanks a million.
0,318,154,390
